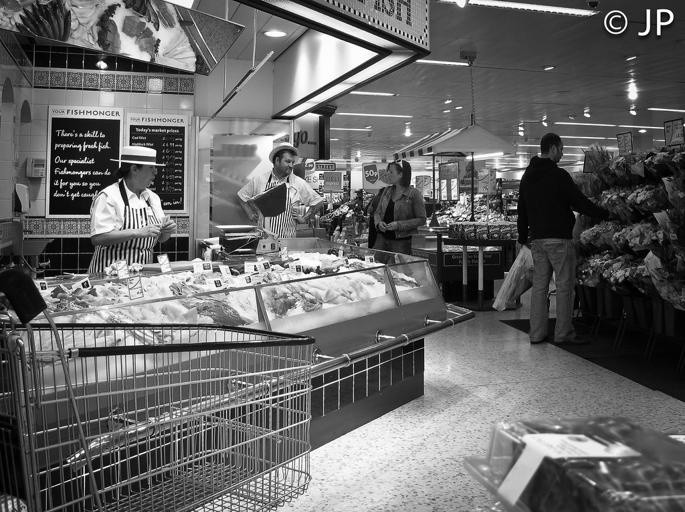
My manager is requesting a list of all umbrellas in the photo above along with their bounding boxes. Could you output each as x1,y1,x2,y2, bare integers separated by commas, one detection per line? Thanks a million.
393,124,516,221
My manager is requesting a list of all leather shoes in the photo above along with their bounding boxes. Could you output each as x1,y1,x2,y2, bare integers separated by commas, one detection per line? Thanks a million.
554,335,589,345
531,335,547,344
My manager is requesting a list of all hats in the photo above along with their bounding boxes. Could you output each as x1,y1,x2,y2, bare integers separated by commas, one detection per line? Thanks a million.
110,146,166,167
268,142,297,162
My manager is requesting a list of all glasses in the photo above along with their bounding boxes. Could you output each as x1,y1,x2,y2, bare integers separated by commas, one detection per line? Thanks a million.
396,159,403,169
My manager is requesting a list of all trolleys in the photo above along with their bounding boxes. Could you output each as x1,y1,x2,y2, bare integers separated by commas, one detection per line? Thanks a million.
0,265,318,512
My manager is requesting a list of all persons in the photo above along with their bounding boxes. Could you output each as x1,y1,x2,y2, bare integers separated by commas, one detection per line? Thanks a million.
372,159,427,255
87,146,177,274
237,142,324,254
517,133,616,346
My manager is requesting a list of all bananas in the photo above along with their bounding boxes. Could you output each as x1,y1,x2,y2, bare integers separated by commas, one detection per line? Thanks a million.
15,0,71,42
